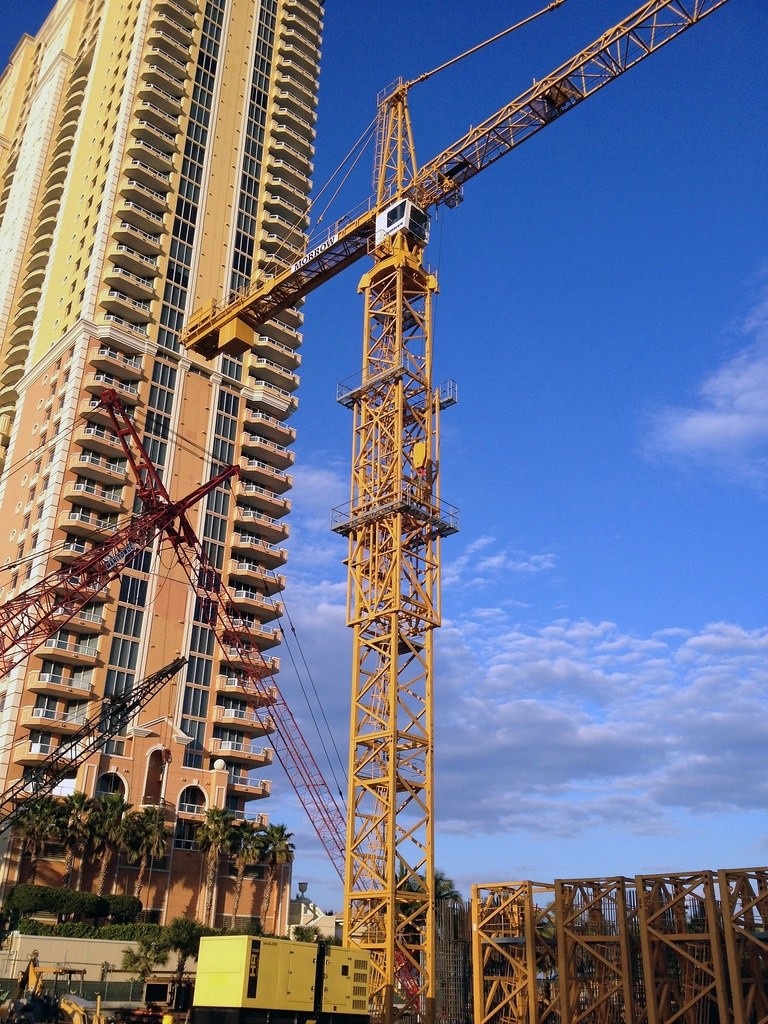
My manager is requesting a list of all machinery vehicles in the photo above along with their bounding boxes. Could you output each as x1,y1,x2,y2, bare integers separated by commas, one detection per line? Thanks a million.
0,947,109,1024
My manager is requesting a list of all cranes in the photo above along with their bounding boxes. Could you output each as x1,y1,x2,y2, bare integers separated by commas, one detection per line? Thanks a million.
0,657,188,838
0,388,419,1017
173,0,768,1024
